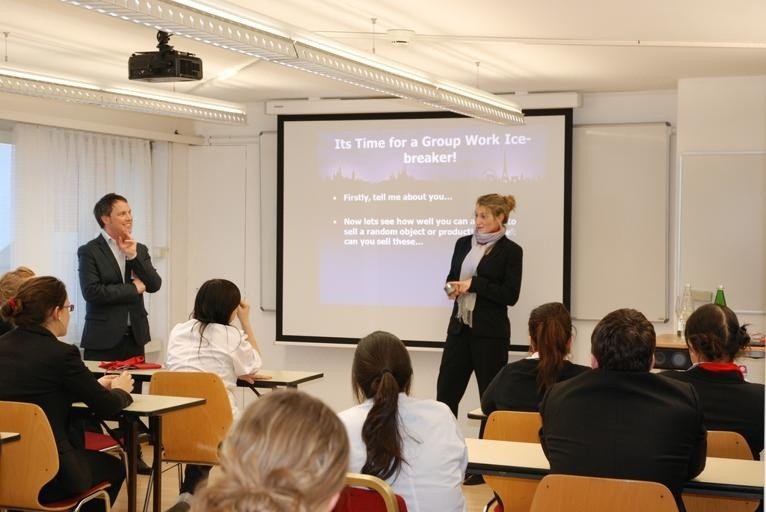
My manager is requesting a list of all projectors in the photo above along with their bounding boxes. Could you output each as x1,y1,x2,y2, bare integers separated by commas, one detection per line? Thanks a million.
128,51,204,83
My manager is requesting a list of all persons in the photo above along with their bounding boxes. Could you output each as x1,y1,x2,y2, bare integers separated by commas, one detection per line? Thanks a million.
77,193,163,476
538,308,708,497
189,390,350,512
481,302,595,417
337,331,467,511
658,304,764,460
163,280,272,511
0,277,134,512
437,193,523,486
0,267,35,336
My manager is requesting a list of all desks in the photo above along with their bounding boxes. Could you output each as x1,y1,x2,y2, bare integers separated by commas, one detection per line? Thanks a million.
470,407,490,418
465,436,764,501
81,360,322,400
72,393,208,510
0,431,22,444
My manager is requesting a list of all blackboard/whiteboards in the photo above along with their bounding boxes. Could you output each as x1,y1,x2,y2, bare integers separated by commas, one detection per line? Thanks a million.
258,122,670,325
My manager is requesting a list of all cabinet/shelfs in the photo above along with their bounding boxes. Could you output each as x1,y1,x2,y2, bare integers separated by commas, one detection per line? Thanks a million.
646,329,766,372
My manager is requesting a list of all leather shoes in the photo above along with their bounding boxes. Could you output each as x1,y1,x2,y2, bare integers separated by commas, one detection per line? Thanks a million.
137,457,149,473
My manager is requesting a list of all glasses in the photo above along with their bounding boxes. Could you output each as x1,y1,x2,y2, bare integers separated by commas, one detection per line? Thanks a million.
59,305,75,313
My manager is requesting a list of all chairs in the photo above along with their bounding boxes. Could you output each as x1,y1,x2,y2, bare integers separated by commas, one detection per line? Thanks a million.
706,431,754,462
481,409,551,512
529,475,677,512
142,371,235,510
337,473,405,512
83,428,129,476
0,399,112,512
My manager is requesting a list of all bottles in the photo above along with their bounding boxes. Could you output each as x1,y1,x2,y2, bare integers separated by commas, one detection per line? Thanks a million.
678,284,693,338
714,285,726,305
